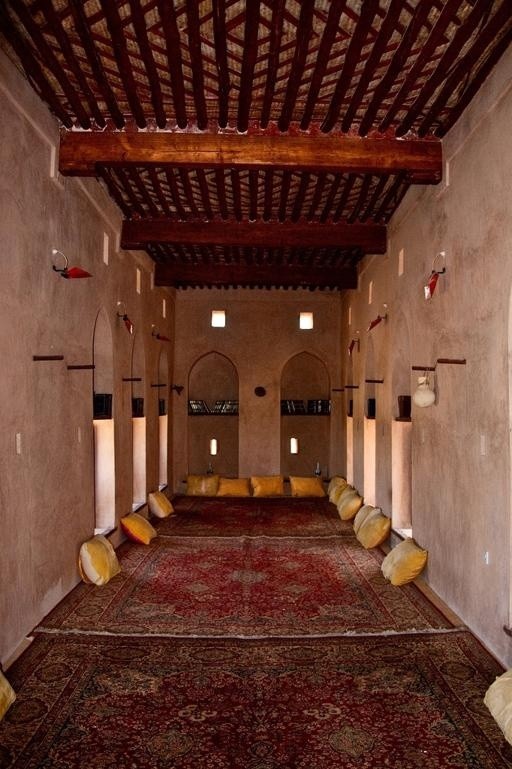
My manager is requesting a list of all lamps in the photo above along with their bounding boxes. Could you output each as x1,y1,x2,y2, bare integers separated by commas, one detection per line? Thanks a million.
117,310,135,335
348,337,359,357
423,267,446,300
152,332,172,342
367,313,387,332
52,249,93,279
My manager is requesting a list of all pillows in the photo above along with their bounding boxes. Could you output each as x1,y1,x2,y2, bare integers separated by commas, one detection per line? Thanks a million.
327,476,346,495
250,476,285,498
352,504,374,535
483,668,512,747
380,537,427,586
288,476,325,498
336,485,364,520
148,491,175,518
215,477,250,497
357,507,390,549
120,511,157,545
184,475,219,497
78,535,122,587
0,671,16,721
329,484,350,505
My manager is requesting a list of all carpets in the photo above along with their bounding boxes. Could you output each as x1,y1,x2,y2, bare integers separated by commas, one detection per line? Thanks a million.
25,535,469,638
